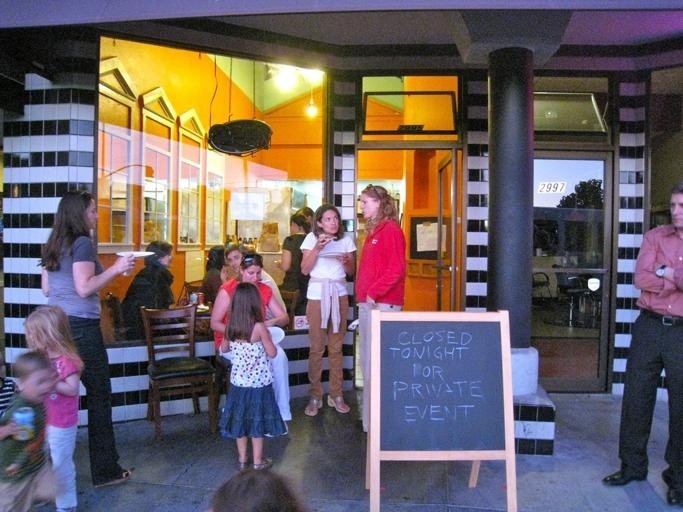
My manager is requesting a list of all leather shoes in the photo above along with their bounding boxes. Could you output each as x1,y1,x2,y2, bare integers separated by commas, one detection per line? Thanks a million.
602,470,634,486
238,456,252,469
92,469,131,488
253,459,272,470
667,488,681,506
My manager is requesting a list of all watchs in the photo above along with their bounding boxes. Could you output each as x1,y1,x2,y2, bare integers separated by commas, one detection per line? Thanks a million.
655,264,666,278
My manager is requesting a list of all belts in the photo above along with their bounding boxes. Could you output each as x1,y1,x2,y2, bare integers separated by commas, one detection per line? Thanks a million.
643,309,683,326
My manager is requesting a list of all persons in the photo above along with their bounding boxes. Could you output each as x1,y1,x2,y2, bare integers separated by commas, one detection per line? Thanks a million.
300,204,351,416
0,352,61,512
201,244,226,304
295,206,315,225
355,184,409,433
210,254,293,439
217,241,288,322
24,305,88,512
219,290,289,471
275,215,311,316
36,193,138,489
267,291,269,293
208,471,299,512
120,239,175,341
601,188,683,508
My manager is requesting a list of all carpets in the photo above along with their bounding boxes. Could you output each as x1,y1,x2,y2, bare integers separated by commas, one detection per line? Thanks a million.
530,336,599,377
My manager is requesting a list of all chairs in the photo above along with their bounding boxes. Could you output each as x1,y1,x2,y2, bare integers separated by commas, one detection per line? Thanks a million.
141,303,219,438
552,264,593,326
105,291,173,342
531,271,552,304
280,290,298,329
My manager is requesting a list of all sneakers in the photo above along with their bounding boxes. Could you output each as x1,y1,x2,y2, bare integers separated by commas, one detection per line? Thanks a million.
305,399,323,416
328,395,350,413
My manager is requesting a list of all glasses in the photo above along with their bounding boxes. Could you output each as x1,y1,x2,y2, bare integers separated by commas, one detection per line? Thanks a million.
367,184,381,201
81,189,91,195
241,256,264,263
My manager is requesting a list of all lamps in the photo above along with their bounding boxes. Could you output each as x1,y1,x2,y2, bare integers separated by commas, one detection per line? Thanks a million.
307,84,318,118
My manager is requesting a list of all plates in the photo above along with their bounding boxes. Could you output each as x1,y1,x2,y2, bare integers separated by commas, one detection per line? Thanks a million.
319,251,345,257
185,304,210,313
266,327,285,346
115,251,156,257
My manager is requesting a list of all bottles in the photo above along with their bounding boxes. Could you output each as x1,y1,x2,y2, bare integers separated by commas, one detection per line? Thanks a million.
14,408,37,441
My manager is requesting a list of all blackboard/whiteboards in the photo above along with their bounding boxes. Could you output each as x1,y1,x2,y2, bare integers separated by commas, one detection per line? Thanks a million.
370,312,515,460
